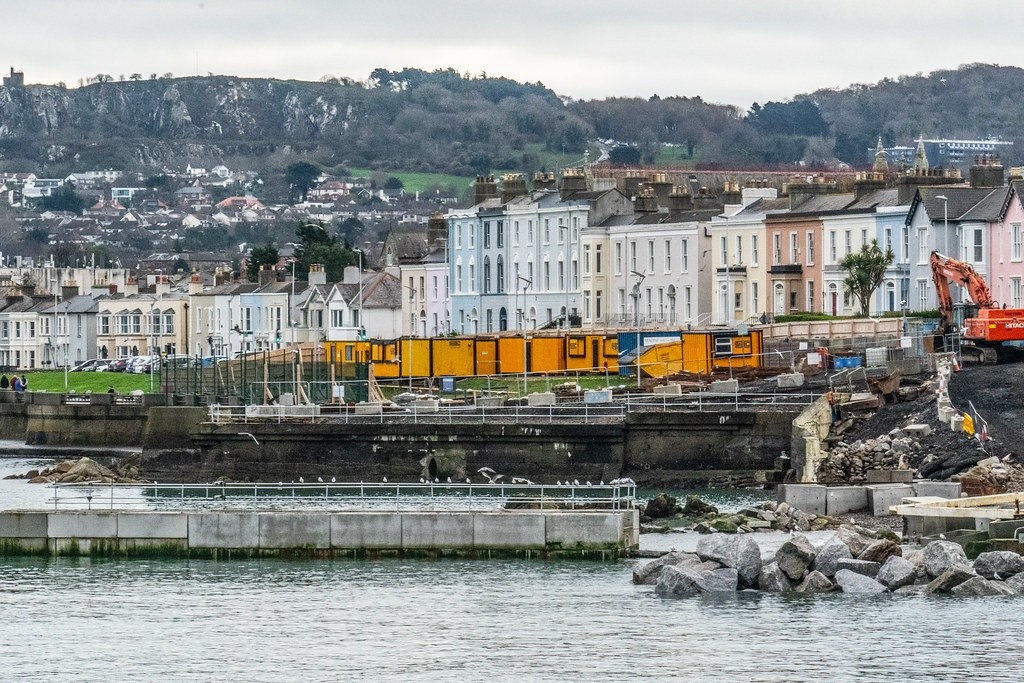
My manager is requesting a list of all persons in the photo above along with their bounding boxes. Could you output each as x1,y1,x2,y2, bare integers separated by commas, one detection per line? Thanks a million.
14,377,25,391
346,398,356,413
0,374,9,389
10,374,19,390
20,375,28,390
107,385,114,393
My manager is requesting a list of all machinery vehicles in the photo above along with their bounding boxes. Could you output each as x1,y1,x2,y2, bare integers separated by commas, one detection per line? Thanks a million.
922,249,1024,364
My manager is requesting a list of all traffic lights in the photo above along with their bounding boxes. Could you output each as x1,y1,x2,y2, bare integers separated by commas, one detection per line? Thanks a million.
358,329,366,340
276,332,280,344
286,260,296,344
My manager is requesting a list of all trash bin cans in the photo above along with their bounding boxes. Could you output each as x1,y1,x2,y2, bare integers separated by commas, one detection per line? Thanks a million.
834,357,861,368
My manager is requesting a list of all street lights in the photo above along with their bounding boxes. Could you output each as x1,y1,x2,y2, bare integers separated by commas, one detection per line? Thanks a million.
51,279,57,370
936,195,948,257
559,225,569,329
154,268,162,361
718,214,732,329
353,248,363,342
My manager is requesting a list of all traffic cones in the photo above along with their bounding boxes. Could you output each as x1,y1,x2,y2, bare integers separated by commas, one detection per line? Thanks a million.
981,425,989,442
952,353,961,373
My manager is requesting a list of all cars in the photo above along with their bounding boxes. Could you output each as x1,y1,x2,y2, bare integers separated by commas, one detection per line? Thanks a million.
69,350,264,374
